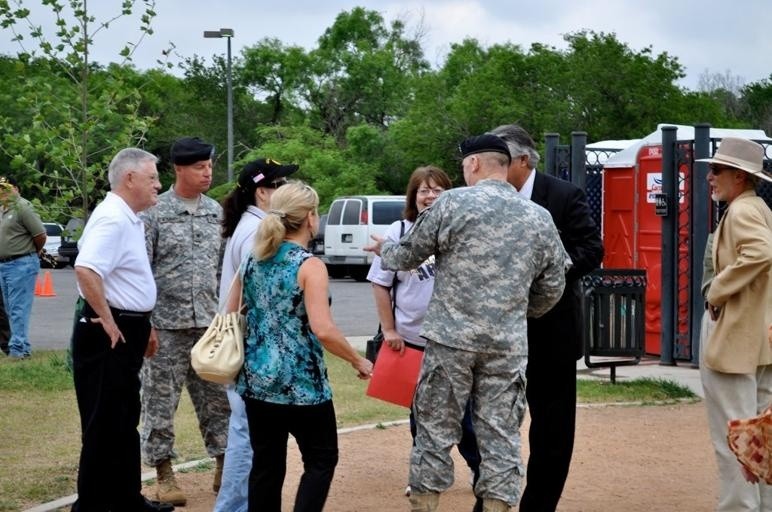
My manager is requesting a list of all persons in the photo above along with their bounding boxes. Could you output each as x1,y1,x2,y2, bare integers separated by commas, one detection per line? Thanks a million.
71,148,174,512
138,136,232,506
367,166,451,498
223,180,375,512
212,157,300,512
692,136,772,512
456,124,603,512
0,176,47,359
362,134,574,512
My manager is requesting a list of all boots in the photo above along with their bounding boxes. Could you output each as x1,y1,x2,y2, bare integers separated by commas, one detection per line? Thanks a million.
211,456,227,493
479,494,511,512
154,457,188,506
407,491,440,512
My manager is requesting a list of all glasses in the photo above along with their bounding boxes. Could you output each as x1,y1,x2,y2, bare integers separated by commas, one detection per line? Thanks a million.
709,163,735,176
415,186,448,197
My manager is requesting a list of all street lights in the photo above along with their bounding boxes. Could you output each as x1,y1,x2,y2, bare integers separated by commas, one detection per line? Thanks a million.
202,25,236,188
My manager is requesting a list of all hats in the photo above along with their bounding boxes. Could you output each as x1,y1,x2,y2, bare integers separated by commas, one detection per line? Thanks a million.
0,176,17,188
238,156,301,191
456,133,512,164
693,136,772,185
171,136,214,167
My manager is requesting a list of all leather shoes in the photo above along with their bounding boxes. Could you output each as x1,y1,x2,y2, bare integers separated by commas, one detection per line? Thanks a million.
139,494,175,512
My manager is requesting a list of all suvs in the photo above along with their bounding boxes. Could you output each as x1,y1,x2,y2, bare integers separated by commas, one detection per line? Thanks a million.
58,214,94,267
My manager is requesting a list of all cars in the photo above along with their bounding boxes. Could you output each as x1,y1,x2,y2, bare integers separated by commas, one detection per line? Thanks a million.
308,214,327,257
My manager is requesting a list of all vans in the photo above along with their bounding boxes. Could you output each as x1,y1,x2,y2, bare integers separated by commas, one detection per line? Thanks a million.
325,196,407,281
42,223,70,264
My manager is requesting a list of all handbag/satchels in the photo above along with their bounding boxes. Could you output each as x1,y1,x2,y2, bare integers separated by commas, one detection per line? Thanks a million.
365,320,394,367
188,312,250,387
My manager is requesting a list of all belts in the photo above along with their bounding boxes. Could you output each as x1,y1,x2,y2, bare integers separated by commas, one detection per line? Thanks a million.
0,252,35,263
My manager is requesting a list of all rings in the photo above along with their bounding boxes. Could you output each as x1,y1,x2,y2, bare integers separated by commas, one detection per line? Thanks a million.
369,373,373,376
